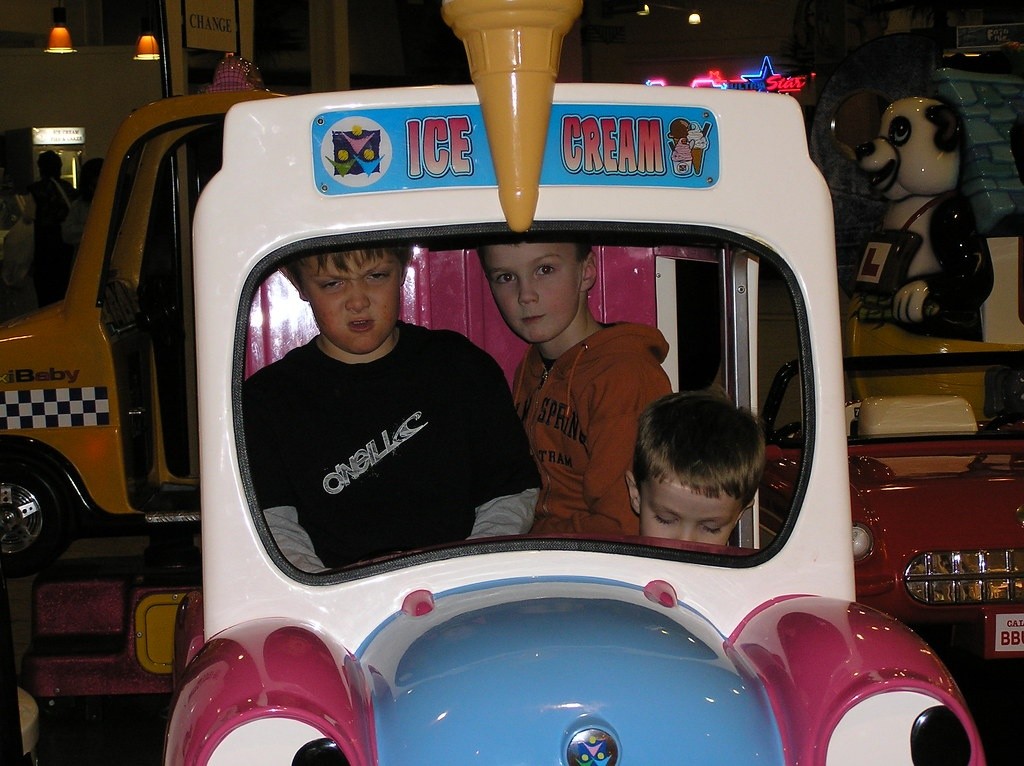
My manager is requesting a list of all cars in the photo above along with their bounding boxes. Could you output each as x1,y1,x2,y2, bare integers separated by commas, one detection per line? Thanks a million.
2,90,204,574
754,349,1024,660
164,87,985,766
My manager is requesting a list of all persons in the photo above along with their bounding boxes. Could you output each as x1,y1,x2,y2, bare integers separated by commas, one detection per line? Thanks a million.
30,150,104,307
242,240,766,577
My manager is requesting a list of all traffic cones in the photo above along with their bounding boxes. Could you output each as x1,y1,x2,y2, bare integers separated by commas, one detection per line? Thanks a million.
433,0,610,231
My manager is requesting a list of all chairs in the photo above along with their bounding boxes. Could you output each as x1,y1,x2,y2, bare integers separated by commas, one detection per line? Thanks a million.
858,394,979,440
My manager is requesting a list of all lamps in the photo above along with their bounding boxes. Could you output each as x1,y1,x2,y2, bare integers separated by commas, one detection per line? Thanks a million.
44,0,78,54
133,0,161,61
